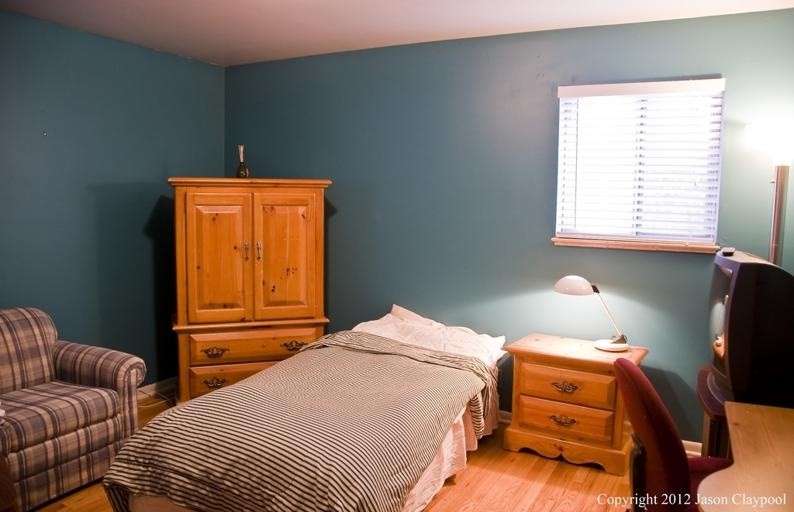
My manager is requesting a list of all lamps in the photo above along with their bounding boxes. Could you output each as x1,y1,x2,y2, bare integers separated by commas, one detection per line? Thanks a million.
554,274,629,352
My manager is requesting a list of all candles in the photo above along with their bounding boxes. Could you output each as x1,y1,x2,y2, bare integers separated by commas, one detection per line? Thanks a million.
238,144,244,162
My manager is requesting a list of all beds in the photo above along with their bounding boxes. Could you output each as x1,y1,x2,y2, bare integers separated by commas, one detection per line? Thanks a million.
102,304,513,511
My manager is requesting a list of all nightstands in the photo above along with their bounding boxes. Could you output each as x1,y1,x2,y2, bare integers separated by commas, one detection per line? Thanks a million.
501,332,649,477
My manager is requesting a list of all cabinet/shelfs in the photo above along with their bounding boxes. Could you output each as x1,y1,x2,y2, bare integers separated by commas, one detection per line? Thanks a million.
697,367,732,457
166,176,333,406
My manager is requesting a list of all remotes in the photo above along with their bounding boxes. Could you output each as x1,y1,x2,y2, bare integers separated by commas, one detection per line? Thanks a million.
723,247,735,256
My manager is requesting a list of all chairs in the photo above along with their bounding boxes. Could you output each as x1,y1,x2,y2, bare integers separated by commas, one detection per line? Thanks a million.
0,306,148,511
613,358,733,512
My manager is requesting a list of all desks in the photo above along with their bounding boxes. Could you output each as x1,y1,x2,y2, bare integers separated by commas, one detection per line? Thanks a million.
697,401,794,512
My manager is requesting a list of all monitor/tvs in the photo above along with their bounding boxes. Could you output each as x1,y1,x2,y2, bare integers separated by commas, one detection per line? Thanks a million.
707,248,794,407
